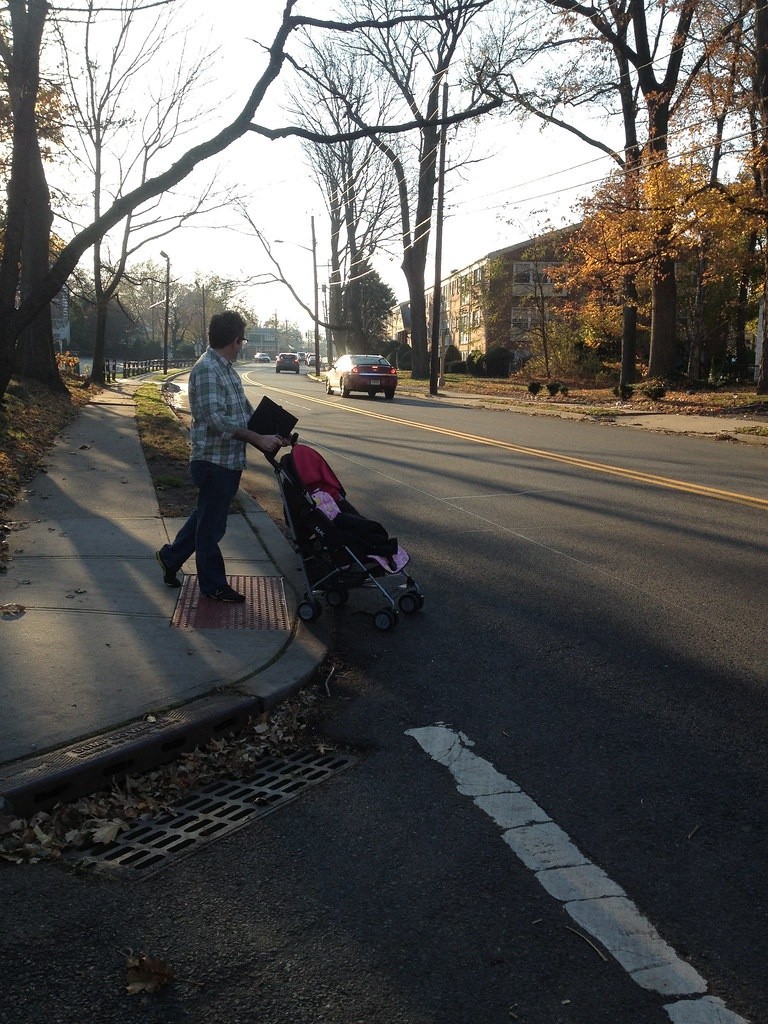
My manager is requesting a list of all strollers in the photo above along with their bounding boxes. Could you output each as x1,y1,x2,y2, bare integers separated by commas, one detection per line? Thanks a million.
264,432,425,632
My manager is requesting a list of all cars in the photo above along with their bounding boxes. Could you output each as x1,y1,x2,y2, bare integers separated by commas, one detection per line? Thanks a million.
254,352,271,363
297,352,322,366
325,354,397,398
275,353,299,374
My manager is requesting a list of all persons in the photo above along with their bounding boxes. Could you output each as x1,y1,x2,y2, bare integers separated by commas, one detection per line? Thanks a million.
155,310,297,604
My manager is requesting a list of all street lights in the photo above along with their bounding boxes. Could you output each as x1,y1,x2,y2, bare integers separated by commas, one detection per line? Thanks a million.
160,250,170,375
275,239,320,376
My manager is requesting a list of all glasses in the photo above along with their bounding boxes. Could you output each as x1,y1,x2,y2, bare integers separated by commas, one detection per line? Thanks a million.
233,336,249,344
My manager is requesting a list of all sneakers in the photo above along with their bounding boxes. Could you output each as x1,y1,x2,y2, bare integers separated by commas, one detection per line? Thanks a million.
200,585,246,604
156,543,180,588
